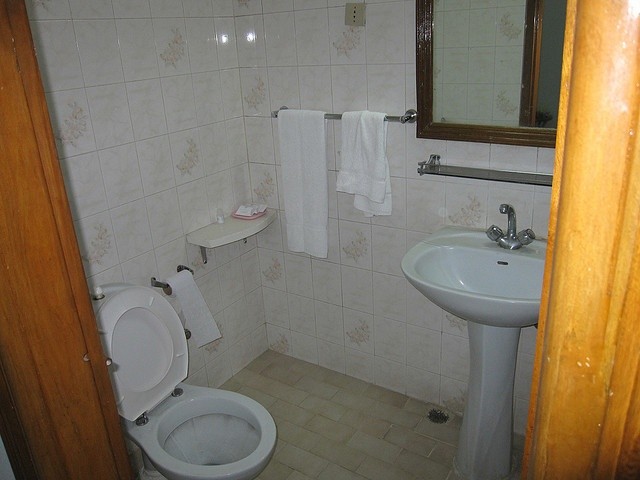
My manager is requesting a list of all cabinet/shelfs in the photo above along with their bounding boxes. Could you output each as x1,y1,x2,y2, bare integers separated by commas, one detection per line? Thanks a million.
186,207,276,250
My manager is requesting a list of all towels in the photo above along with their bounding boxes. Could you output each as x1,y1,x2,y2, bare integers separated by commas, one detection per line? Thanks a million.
278,109,328,259
337,111,392,217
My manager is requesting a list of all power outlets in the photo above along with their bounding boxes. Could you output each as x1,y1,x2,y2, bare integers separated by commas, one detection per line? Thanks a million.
345,2,364,26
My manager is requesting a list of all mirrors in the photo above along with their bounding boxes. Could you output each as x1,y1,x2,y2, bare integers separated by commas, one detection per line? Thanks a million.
416,0,566,149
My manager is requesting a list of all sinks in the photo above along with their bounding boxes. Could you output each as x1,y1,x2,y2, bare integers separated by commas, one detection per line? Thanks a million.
400,225,545,328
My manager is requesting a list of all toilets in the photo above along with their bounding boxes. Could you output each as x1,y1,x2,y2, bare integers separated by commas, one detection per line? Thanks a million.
93,283,277,480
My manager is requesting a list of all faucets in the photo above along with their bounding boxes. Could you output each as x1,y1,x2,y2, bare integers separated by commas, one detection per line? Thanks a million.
499,203,517,240
